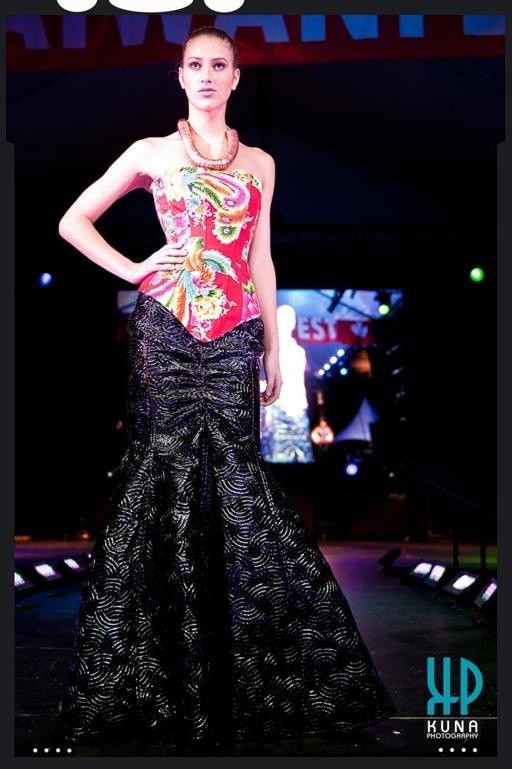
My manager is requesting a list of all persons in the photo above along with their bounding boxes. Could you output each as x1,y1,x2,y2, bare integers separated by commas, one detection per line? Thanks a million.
53,27,398,744
259,304,314,464
310,346,379,465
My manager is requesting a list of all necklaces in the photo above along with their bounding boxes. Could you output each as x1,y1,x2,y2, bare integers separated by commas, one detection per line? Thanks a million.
177,118,239,171
